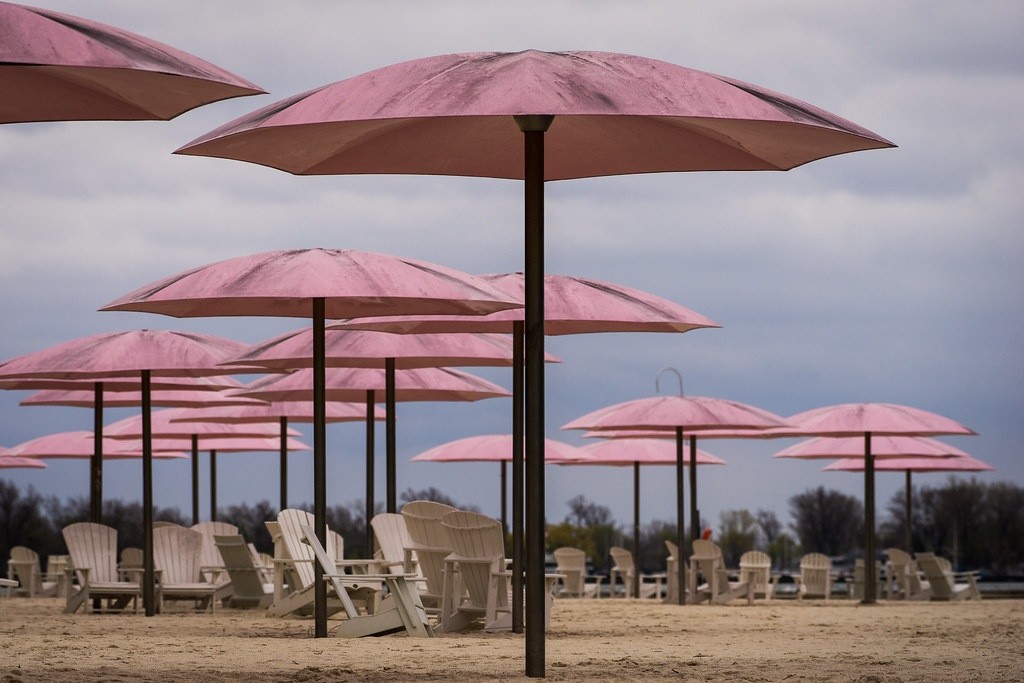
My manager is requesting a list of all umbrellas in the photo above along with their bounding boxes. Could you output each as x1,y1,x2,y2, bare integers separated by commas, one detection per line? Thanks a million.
0,321,991,638
95,246,525,638
169,49,897,675
327,270,726,631
0,0,269,124
561,395,800,604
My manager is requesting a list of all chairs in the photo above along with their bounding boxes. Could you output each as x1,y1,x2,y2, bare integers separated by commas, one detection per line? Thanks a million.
0,500,981,639
